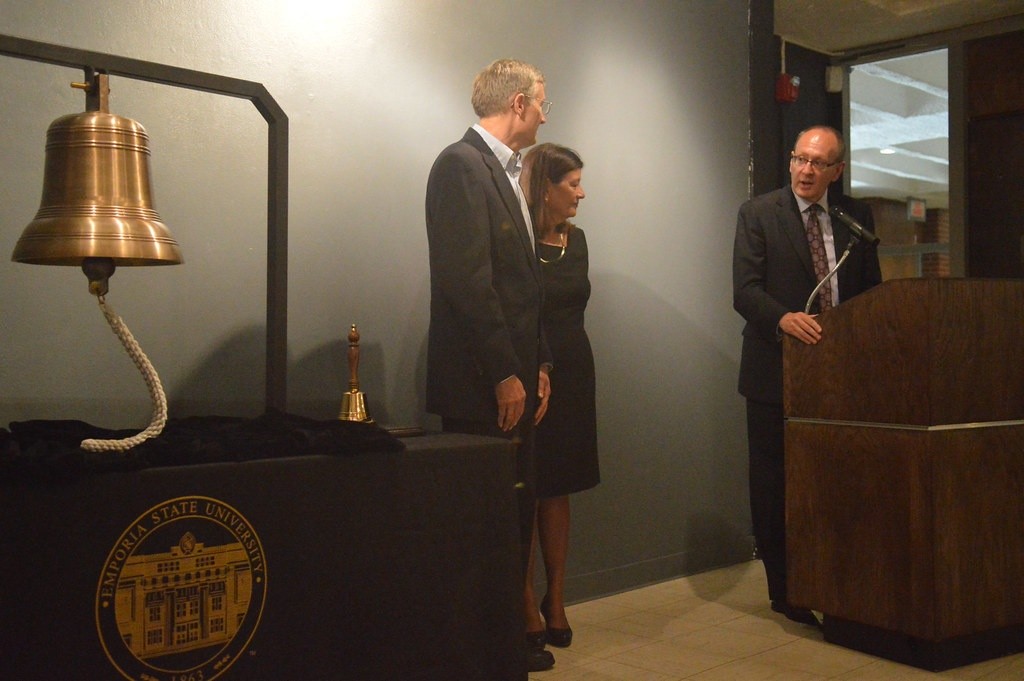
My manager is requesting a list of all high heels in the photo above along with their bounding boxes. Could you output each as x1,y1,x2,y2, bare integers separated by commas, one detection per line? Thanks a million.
539,595,573,648
522,607,547,647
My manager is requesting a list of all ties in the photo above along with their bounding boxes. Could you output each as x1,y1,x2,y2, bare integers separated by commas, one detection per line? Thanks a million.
803,206,833,315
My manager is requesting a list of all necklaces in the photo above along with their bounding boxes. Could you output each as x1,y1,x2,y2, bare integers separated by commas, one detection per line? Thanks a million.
540,233,565,264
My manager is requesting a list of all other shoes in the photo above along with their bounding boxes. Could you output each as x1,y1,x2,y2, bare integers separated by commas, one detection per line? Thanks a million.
521,645,556,672
770,596,818,627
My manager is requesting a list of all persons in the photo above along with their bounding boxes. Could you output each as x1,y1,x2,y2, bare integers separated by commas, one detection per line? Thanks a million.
733,127,883,622
426,60,556,671
519,144,600,647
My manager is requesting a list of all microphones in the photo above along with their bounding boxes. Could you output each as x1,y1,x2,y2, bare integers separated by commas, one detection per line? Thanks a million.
828,205,880,248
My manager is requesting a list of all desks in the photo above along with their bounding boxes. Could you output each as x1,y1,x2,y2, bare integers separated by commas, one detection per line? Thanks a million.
0,432,515,681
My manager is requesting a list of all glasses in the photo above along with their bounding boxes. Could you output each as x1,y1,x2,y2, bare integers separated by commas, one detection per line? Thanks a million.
792,151,842,172
509,94,553,115
